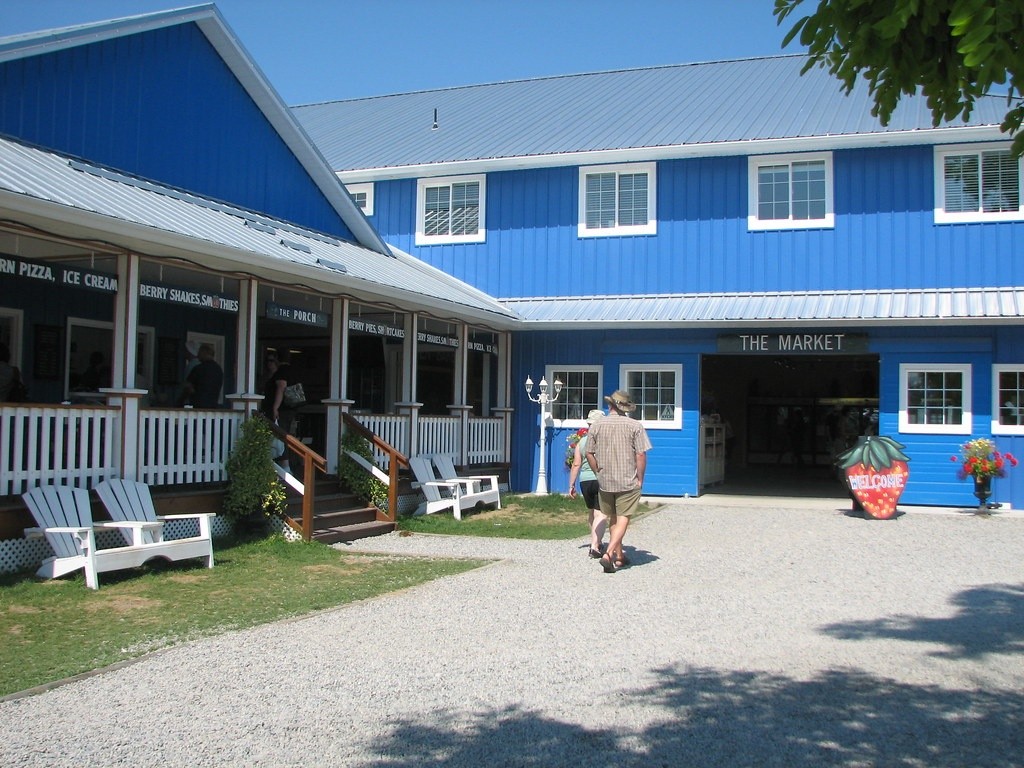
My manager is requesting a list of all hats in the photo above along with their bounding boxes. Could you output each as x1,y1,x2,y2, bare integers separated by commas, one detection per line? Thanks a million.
585,409,606,424
605,390,636,413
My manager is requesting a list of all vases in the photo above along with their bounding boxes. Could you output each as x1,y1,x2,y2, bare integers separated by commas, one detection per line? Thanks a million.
972,474,992,516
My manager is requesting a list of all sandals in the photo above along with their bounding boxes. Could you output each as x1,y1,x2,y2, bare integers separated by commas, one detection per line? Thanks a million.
614,557,630,567
600,553,616,573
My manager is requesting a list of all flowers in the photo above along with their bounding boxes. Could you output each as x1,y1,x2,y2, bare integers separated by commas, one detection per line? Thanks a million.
564,427,588,470
950,436,1019,481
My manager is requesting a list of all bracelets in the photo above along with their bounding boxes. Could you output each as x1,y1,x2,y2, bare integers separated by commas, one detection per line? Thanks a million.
570,485,575,489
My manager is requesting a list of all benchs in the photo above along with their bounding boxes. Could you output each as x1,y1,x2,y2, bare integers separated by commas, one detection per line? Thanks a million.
408,454,502,522
19,478,216,590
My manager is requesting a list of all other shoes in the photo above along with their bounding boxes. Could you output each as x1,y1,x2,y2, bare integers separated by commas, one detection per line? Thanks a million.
589,549,603,558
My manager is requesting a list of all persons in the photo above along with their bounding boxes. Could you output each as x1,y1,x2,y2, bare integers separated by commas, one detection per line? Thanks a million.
585,389,653,573
569,410,608,556
720,404,875,482
1001,396,1018,424
0,340,306,474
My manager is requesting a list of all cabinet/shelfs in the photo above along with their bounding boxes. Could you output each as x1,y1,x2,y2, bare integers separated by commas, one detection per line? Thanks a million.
700,424,726,490
